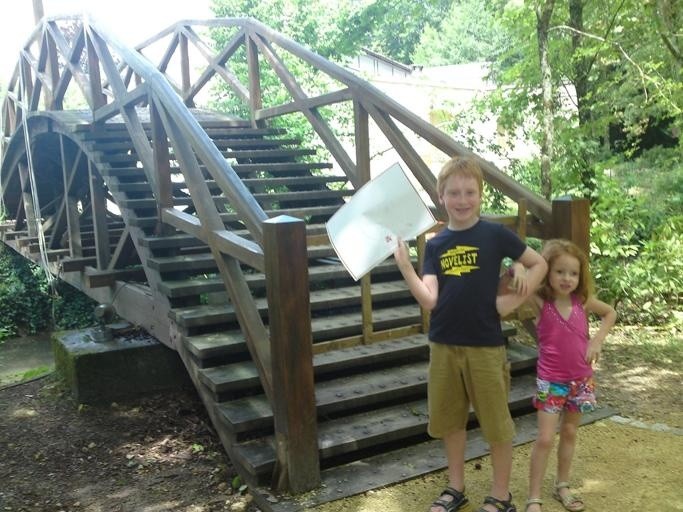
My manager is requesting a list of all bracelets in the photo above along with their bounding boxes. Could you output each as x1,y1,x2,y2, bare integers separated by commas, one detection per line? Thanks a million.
508,268,514,278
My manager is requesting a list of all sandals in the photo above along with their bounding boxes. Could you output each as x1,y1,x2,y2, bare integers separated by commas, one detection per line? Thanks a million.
523,496,543,512
428,487,469,512
476,491,517,511
550,480,586,511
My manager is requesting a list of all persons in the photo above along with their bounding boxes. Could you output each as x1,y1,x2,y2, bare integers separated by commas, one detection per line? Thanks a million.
497,236,620,512
392,157,551,512
